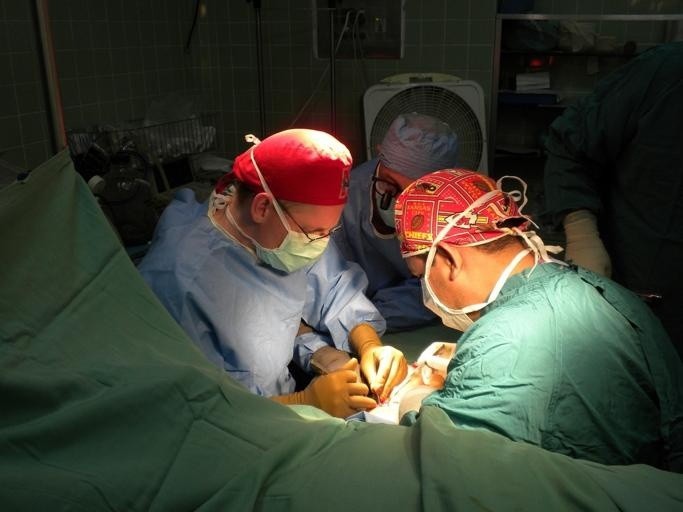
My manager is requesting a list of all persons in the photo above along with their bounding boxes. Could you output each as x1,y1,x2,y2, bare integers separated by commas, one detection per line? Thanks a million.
333,111,460,331
542,41,683,359
394,168,683,475
137,128,408,420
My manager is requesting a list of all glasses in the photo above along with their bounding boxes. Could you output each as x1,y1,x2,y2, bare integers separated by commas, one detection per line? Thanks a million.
276,200,346,242
372,160,403,199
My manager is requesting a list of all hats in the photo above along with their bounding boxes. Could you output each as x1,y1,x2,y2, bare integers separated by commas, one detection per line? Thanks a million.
379,113,460,181
233,126,353,208
394,168,532,259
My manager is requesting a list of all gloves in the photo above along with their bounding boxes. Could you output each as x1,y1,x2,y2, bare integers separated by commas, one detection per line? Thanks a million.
270,369,377,418
415,341,456,385
348,323,407,403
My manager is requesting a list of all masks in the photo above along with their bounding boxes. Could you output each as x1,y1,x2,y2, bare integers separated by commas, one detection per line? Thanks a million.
374,184,396,228
420,277,475,331
252,217,331,273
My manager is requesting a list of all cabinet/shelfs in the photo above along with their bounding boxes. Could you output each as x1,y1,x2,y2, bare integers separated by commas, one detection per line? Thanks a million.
492,11,682,245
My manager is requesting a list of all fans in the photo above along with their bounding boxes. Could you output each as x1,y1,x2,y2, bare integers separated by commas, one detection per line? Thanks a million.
362,71,489,185
